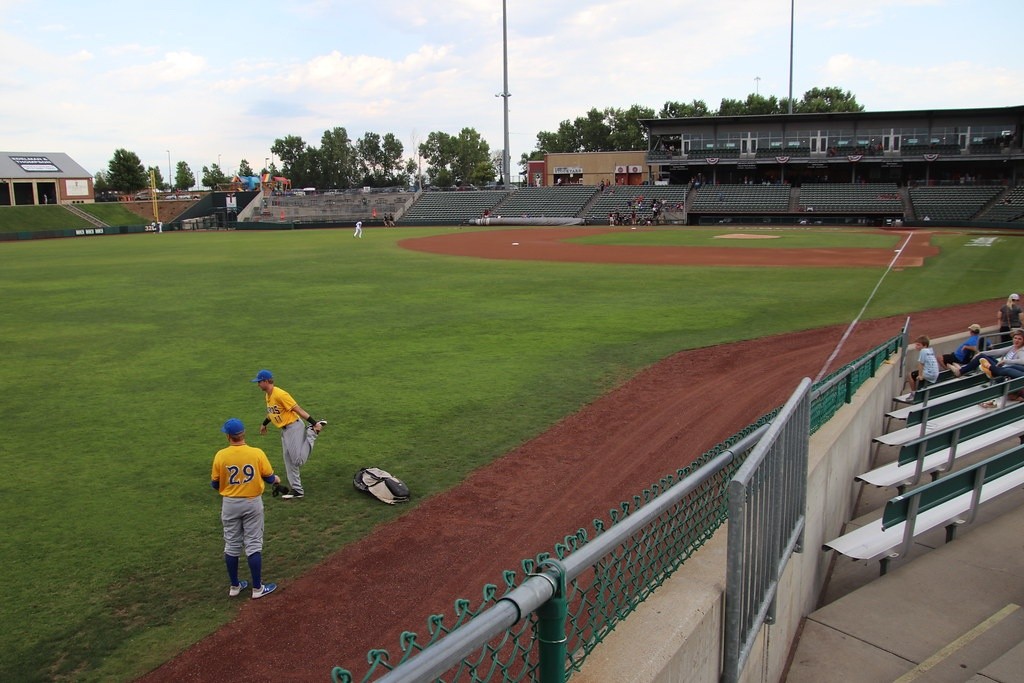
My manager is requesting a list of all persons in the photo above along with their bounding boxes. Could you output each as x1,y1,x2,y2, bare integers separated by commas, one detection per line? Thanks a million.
354,221,362,238
998,293,1024,342
44,194,47,204
151,220,164,234
483,209,490,218
176,188,179,200
251,370,327,499
372,208,395,226
211,417,281,598
826,142,884,157
744,174,789,186
558,176,569,186
660,144,681,161
609,195,683,227
535,174,542,189
906,335,939,401
947,329,1024,400
600,177,624,195
687,175,721,189
522,212,530,219
280,211,285,222
929,142,937,150
937,324,993,370
799,172,1009,186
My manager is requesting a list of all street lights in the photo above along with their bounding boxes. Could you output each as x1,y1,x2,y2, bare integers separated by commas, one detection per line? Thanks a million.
265,157,269,170
218,154,221,167
496,92,513,188
197,171,200,190
167,151,171,186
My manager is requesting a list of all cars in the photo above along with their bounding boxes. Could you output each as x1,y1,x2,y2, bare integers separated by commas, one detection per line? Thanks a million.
135,192,150,200
192,194,201,199
178,194,191,199
164,194,176,200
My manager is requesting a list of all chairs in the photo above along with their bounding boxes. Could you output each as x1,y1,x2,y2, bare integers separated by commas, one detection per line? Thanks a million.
243,182,1024,227
644,144,1024,158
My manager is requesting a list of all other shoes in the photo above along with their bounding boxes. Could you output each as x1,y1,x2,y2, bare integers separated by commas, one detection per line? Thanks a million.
947,362,961,377
906,392,915,400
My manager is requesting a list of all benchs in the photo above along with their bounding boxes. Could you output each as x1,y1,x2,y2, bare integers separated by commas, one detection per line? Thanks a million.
822,356,1024,578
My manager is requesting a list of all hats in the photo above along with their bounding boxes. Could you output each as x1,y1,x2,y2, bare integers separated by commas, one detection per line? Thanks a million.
1010,294,1019,299
251,370,272,382
968,324,980,332
221,418,244,434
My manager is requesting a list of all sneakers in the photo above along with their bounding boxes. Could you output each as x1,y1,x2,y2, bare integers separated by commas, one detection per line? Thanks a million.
979,358,991,369
281,489,304,499
979,365,992,379
252,583,277,599
229,580,248,597
305,421,327,434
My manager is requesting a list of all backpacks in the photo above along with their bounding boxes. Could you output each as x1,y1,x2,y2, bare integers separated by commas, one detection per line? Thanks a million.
352,466,410,505
